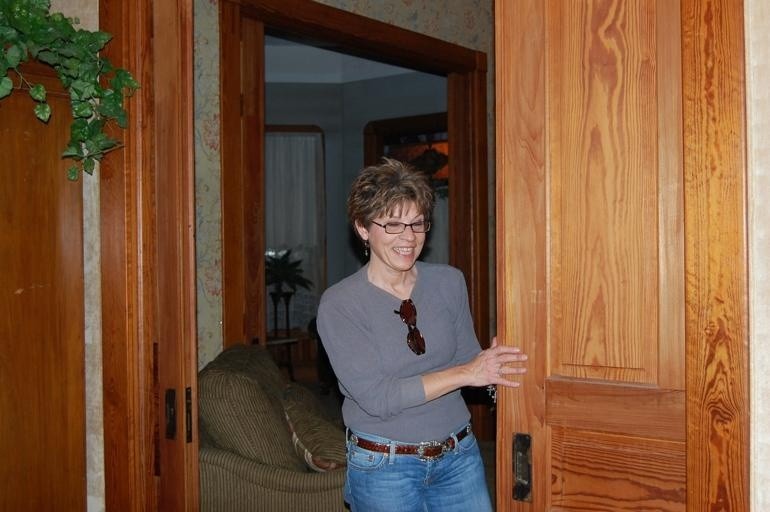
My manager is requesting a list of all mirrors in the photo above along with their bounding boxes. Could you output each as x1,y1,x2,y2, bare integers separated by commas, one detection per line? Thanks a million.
259,124,328,350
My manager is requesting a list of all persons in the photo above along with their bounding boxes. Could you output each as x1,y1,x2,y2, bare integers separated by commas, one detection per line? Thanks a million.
313,156,529,512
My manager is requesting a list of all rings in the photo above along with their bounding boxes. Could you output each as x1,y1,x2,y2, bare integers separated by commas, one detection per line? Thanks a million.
499,367,503,375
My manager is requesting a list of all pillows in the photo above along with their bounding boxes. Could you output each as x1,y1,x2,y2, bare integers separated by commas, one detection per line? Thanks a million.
278,379,346,473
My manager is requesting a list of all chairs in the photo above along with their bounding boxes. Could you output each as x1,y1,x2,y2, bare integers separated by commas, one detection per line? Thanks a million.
198,343,348,511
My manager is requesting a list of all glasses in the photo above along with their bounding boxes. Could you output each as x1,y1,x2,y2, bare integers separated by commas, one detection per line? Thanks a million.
392,297,426,356
371,219,431,234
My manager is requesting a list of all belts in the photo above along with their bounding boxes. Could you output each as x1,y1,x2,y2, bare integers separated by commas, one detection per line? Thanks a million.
347,426,469,460
